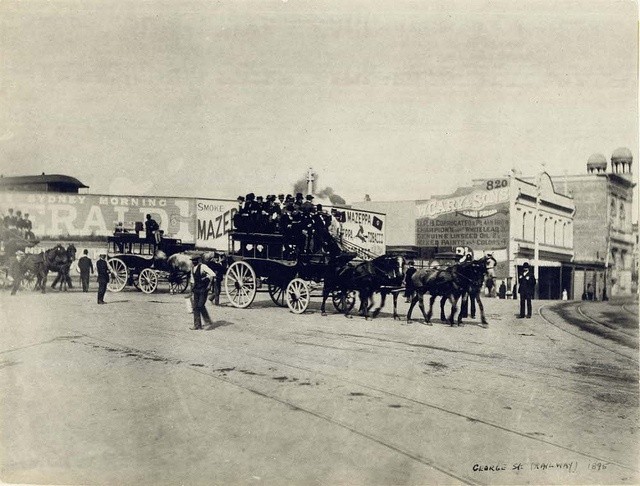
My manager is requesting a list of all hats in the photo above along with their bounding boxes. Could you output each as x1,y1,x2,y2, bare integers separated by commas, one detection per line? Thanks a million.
306,195,314,198
271,195,276,198
296,192,303,198
522,263,531,268
257,196,262,199
279,194,284,199
190,254,201,260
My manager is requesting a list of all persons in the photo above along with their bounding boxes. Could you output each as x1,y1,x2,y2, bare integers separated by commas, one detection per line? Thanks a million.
188,252,216,330
209,253,224,306
15,209,24,232
112,220,129,253
96,251,111,304
5,206,16,232
516,262,536,318
24,212,33,233
499,280,506,298
403,261,418,304
145,212,159,242
485,275,495,297
78,249,94,292
237,193,342,255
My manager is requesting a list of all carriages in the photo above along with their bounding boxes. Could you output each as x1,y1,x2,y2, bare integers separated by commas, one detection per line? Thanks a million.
1,216,76,295
105,221,225,294
224,225,497,328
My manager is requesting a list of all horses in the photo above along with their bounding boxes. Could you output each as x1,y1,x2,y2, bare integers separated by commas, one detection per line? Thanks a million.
320,252,395,320
29,242,77,291
358,254,406,321
427,249,497,328
167,250,227,294
10,243,68,295
406,255,484,327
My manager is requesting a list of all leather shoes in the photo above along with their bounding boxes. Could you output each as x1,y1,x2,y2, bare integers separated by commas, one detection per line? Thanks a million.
203,322,214,330
517,313,523,317
526,313,531,317
188,323,201,331
